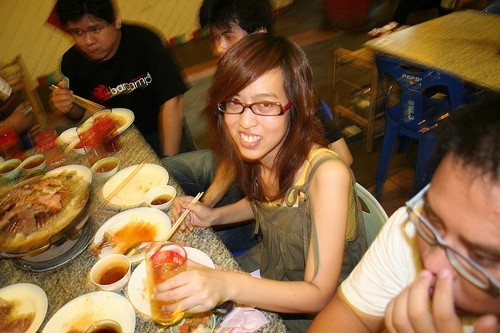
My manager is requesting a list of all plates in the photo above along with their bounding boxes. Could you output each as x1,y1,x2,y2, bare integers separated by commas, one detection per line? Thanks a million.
94,208,171,263
0,283,48,333
81,107,135,137
168,311,217,333
41,290,137,333
45,164,93,184
103,163,169,206
128,246,216,316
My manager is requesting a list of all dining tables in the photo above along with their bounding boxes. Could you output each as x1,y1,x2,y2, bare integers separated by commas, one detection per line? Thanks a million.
0,125,288,333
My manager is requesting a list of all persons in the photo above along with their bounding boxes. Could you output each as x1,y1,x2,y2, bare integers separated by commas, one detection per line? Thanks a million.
0,103,33,157
172,32,368,333
313,91,500,333
198,0,354,168
50,0,192,157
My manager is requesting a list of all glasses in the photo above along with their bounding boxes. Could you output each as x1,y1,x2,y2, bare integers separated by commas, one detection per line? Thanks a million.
217,99,292,116
405,184,500,298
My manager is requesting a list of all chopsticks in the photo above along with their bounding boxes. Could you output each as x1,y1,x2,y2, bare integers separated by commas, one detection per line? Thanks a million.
97,161,148,208
151,191,203,252
49,83,105,109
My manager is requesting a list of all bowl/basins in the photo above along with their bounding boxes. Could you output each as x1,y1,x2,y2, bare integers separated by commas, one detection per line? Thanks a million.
89,254,132,292
69,138,90,156
0,154,120,181
144,184,177,209
60,126,82,145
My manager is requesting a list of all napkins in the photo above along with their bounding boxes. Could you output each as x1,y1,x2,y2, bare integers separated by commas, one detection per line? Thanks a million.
219,269,269,329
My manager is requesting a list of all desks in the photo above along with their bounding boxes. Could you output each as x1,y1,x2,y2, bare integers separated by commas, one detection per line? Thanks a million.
364,8,500,94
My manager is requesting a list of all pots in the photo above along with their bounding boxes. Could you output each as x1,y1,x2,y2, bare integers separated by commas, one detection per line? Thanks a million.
0,173,93,259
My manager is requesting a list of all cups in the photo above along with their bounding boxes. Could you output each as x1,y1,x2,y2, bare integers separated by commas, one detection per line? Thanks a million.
76,108,121,166
1,121,27,162
34,126,68,167
144,240,188,326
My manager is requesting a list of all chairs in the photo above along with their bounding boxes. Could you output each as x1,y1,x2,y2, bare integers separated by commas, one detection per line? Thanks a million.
374,55,466,201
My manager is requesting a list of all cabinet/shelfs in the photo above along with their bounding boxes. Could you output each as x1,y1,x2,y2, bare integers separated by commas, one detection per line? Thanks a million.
333,47,403,155
0,54,49,137
